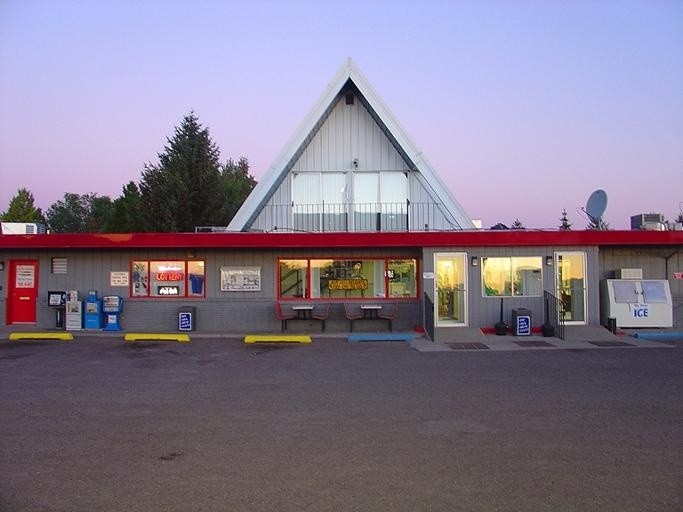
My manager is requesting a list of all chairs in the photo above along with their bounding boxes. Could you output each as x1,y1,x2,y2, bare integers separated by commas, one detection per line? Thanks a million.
342,301,363,333
377,300,399,333
313,299,334,333
272,299,294,333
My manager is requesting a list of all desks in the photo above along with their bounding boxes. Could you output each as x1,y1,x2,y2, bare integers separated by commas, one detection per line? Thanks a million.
290,303,315,318
360,303,383,319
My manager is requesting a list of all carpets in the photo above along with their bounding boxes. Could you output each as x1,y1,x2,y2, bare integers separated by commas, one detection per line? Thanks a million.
512,340,554,346
449,341,489,349
587,340,635,347
